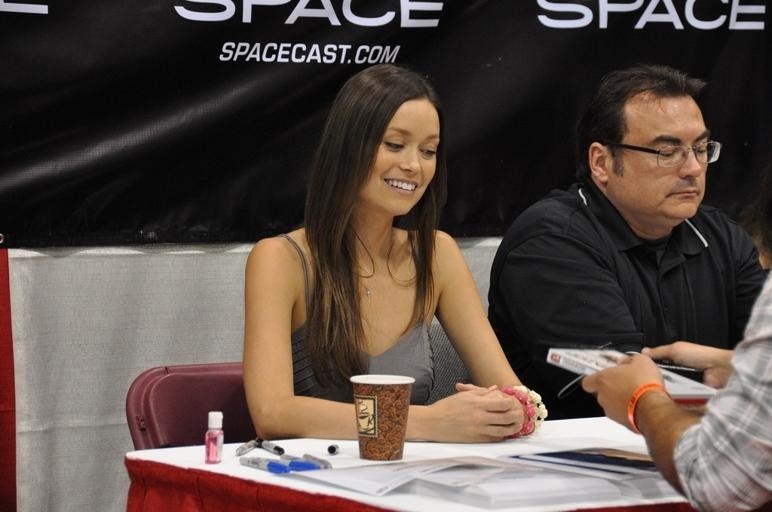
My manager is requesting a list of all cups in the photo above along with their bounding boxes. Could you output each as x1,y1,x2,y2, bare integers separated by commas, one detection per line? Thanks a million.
349,374,415,462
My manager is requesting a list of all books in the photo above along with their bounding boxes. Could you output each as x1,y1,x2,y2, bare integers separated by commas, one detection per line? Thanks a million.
543,346,719,410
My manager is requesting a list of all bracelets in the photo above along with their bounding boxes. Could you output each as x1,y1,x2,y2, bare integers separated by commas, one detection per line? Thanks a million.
513,384,548,436
626,382,673,434
499,386,536,440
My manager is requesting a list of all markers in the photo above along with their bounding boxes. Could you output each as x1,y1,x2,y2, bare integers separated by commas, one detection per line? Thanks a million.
236,437,339,474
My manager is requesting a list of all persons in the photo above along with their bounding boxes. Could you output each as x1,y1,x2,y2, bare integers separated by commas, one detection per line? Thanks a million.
582,264,771,512
487,66,767,421
241,62,548,446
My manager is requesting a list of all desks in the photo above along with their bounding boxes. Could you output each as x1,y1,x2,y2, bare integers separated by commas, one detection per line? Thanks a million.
125,413,695,512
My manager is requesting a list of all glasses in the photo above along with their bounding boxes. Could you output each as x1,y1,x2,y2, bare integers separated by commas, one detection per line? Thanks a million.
601,140,722,168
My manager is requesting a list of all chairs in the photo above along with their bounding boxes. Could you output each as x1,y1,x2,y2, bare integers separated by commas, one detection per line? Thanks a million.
125,361,253,450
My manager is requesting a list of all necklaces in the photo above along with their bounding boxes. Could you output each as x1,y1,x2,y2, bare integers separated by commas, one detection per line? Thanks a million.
358,276,373,296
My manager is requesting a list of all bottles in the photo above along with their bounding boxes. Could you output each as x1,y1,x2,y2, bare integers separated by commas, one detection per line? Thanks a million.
204,411,225,463
